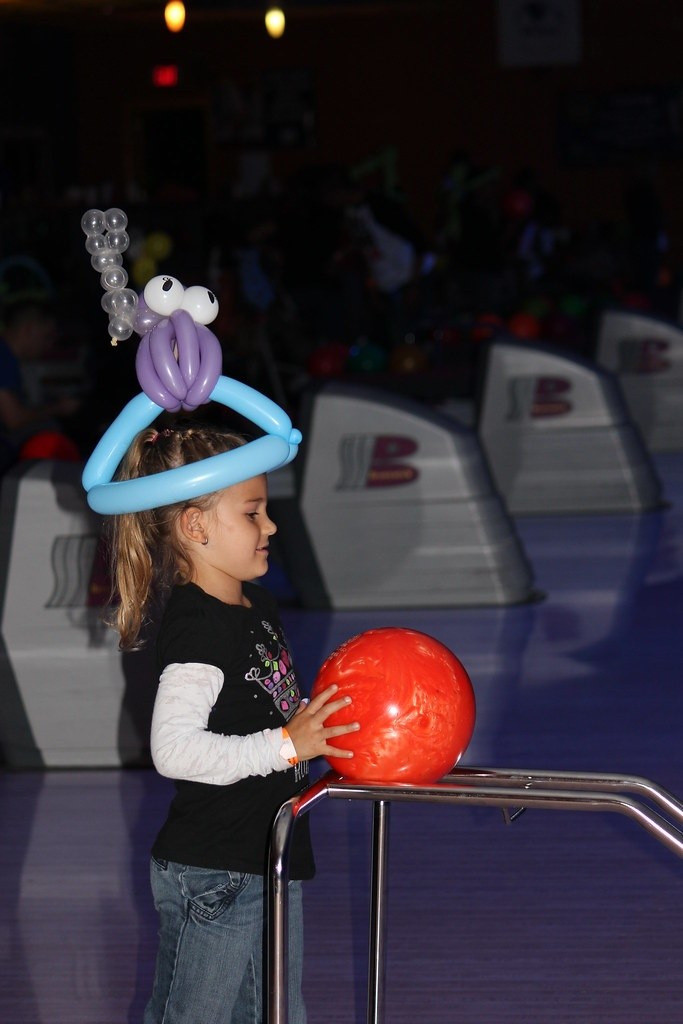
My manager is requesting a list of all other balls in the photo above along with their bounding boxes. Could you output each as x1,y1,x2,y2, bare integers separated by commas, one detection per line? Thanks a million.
312,629,474,786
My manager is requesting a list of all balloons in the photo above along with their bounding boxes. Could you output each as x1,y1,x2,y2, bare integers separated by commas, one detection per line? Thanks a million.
80,208,302,515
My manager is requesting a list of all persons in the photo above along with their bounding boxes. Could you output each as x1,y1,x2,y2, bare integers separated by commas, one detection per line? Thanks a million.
1,299,81,478
4,135,683,430
103,428,364,1023
